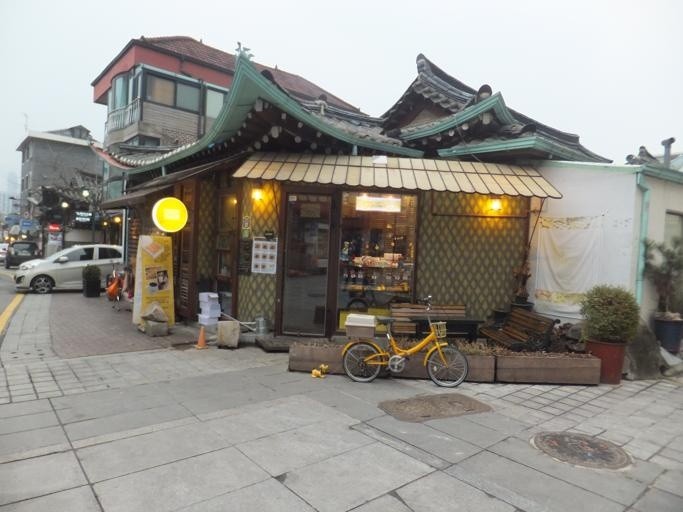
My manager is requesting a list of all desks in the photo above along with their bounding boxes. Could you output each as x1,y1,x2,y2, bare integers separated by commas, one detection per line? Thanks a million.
408,315,485,343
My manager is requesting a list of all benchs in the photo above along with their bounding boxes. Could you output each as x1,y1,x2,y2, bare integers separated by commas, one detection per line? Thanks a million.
479,309,560,352
391,303,466,340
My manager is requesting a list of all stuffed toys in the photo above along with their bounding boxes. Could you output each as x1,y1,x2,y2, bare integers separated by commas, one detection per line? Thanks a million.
310,363,328,377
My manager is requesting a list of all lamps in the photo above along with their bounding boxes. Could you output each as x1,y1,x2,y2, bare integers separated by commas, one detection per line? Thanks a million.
489,196,504,214
250,180,266,200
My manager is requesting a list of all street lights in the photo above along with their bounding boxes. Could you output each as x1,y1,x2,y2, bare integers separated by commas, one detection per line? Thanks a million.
103,222,107,243
114,217,120,244
61,202,68,248
9,197,22,200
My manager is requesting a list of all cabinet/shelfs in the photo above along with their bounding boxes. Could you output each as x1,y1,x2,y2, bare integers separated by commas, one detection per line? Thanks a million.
305,222,330,270
344,263,414,306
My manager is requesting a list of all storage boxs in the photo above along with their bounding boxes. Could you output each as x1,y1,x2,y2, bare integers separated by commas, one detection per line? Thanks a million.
345,314,375,339
197,292,221,325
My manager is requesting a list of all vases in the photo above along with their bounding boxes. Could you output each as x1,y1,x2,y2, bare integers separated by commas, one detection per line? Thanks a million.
492,308,509,323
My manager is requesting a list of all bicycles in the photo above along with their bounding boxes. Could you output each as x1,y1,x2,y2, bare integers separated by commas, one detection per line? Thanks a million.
341,295,468,388
347,274,411,309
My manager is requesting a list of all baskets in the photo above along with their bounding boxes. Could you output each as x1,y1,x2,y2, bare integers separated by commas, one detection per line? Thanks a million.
431,322,447,339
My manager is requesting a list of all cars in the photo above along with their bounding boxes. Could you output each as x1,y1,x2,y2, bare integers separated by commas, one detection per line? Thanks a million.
14,244,122,294
0,241,42,269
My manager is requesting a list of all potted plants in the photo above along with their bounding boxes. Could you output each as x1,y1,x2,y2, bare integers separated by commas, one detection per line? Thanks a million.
514,287,528,303
641,234,683,354
511,301,534,312
579,284,641,385
287,338,383,378
391,336,496,383
492,347,602,385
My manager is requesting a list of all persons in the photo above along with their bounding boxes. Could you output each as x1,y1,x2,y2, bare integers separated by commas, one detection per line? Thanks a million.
158,271,168,289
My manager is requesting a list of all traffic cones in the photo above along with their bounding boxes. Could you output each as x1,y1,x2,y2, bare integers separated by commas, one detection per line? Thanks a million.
194,326,208,349
